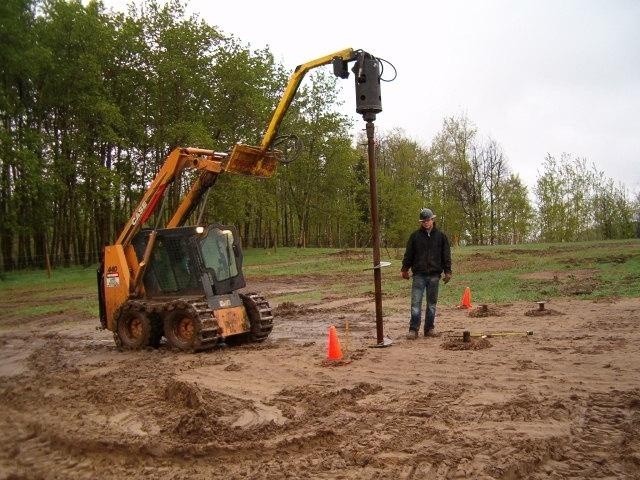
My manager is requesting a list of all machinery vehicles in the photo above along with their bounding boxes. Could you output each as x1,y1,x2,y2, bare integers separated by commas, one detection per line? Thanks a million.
95,46,396,355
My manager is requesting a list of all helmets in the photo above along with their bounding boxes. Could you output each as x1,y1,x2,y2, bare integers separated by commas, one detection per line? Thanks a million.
419,208,436,223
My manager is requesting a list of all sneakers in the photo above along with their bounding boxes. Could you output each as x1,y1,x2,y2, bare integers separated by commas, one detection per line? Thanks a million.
407,329,418,338
424,328,439,336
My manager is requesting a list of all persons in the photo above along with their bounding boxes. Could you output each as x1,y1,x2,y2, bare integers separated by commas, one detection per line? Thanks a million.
400,207,453,339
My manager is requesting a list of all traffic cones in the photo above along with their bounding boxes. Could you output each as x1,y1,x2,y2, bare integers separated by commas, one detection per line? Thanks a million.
327,325,345,361
462,286,473,309
